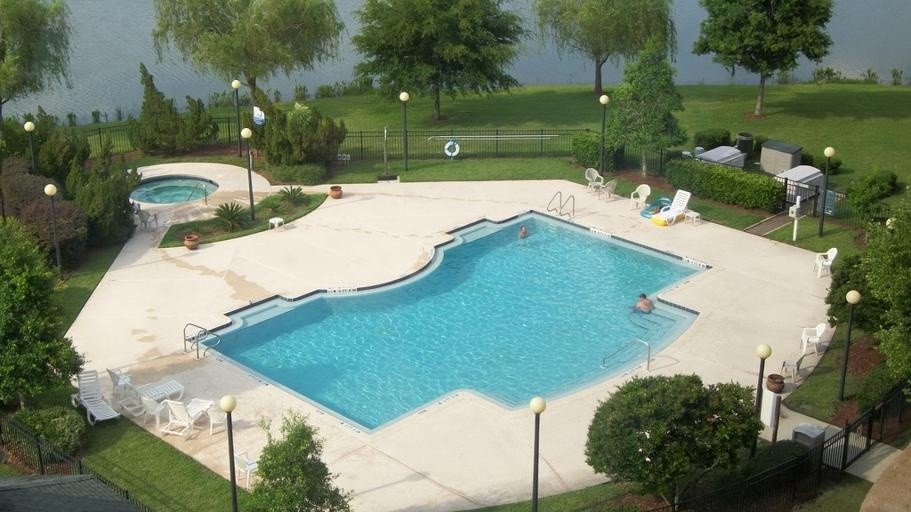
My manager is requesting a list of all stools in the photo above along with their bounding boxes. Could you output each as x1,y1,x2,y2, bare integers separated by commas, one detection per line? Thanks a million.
268,217,285,232
685,211,700,225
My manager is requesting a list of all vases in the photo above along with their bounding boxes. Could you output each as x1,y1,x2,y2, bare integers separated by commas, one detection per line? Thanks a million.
330,186,342,199
184,234,200,250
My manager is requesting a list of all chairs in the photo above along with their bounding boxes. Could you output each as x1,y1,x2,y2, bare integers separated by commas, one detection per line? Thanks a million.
630,183,691,226
137,210,159,231
810,246,837,279
781,322,827,384
70,366,227,443
585,167,618,201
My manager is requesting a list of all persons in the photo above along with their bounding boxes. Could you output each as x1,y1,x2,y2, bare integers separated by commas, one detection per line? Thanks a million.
630,292,655,314
517,225,530,239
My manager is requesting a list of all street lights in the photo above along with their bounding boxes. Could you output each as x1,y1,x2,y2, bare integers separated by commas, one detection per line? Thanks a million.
597,94,611,178
231,79,243,159
398,91,412,174
750,344,772,461
24,121,37,175
817,147,835,238
838,290,862,402
529,396,546,512
44,183,66,284
218,394,239,511
241,128,256,223
